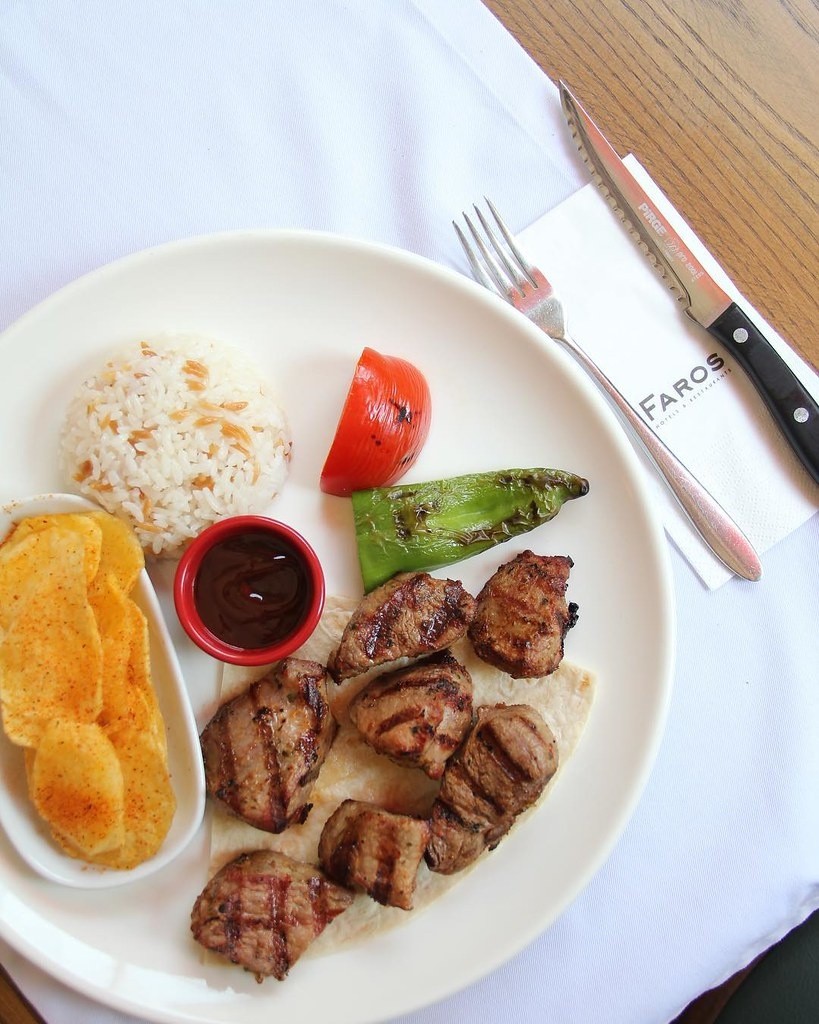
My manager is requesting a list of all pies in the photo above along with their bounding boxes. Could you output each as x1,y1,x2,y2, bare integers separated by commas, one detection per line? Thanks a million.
210,592,598,953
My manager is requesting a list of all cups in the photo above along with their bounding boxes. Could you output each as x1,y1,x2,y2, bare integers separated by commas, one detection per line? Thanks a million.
174,515,326,667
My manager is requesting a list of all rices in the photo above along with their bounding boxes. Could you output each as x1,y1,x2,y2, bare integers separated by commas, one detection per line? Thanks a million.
56,331,292,562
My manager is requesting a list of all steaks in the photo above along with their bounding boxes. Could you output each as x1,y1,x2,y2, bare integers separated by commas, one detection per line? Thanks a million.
189,850,356,984
200,658,341,835
422,702,559,876
347,647,474,779
332,571,476,685
466,550,580,680
319,798,433,910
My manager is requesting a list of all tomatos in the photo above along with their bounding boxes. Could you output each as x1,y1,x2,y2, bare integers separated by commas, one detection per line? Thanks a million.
320,347,431,497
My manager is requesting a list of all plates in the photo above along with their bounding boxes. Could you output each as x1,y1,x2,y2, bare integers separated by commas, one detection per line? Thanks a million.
0,493,205,889
0,226,675,1024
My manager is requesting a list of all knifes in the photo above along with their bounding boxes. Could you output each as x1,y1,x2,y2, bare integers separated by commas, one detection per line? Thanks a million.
556,79,818,485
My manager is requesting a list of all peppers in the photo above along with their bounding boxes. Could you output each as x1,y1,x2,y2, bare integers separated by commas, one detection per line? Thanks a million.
350,468,589,592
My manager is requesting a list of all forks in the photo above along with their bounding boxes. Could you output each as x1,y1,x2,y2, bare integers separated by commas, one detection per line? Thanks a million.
451,195,763,583
